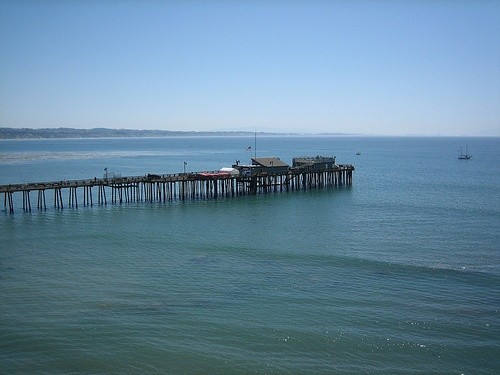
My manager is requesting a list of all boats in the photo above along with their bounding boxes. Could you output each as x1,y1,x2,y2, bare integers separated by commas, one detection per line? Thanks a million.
355,152,361,155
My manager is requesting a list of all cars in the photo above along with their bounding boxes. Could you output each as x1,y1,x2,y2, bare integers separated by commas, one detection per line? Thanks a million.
147,174,162,180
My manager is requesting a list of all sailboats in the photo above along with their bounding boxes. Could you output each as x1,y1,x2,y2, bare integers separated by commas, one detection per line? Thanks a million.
457,143,473,159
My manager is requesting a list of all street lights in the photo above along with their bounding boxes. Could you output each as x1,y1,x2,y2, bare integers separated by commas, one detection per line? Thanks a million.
184,161,188,175
104,167,108,178
